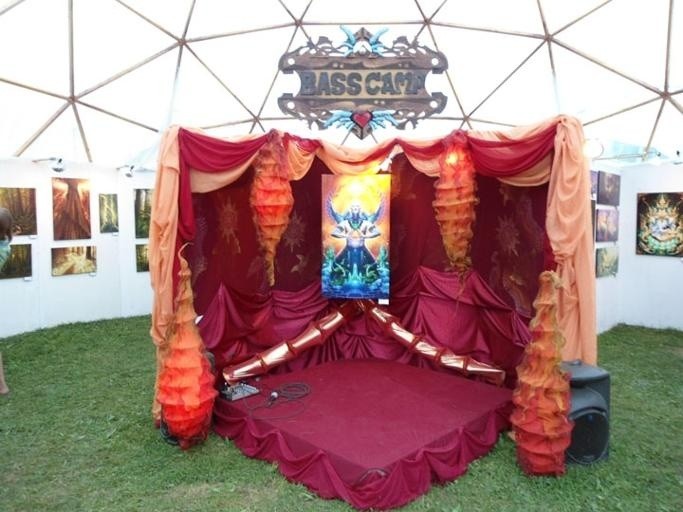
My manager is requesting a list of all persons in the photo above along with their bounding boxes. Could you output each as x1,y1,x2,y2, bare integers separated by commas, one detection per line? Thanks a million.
0,208,16,393
331,201,382,274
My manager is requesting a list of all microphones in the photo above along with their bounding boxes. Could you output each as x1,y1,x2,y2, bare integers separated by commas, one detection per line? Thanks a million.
265,391,279,409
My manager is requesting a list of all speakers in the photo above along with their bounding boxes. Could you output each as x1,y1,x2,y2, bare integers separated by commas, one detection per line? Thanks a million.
550,358,614,467
